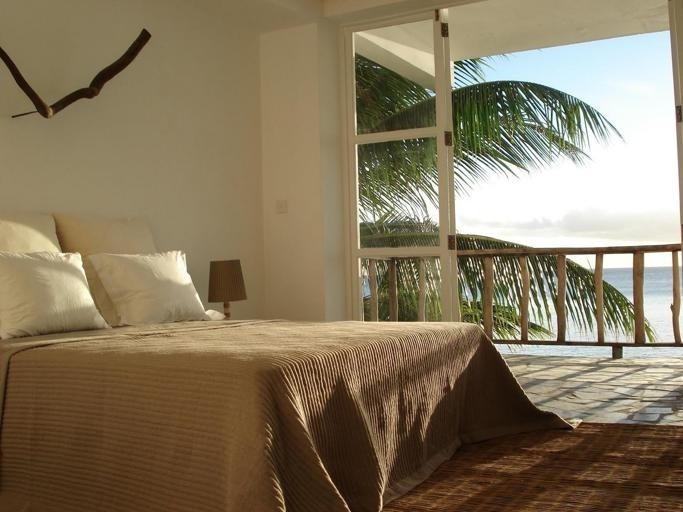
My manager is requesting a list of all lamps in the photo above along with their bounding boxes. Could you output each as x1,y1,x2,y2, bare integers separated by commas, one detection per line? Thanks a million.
208,258,248,320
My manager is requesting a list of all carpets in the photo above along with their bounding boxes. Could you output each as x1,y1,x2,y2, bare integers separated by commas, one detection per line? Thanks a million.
383,421,683,511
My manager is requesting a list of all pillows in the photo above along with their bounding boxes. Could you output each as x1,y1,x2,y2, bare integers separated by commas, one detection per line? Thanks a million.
0,208,212,341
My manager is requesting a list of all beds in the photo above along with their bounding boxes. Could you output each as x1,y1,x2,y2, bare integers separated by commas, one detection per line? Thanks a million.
0,318,578,511
